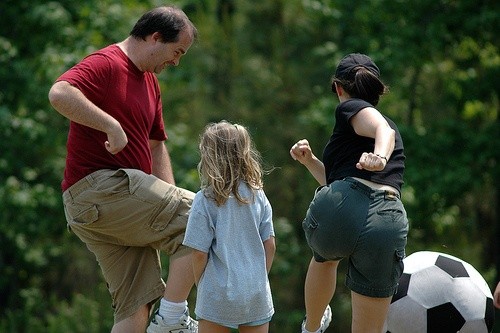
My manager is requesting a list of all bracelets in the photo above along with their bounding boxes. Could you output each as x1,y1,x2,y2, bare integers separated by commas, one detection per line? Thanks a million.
376,154,388,163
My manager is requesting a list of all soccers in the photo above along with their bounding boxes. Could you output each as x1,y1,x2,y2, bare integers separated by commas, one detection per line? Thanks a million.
384,251,496,333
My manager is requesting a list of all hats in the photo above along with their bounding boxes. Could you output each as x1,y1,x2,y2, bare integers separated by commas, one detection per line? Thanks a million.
335,53,379,79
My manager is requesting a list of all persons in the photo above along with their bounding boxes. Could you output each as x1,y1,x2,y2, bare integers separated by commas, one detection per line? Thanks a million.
290,53,409,333
49,6,209,333
182,120,276,333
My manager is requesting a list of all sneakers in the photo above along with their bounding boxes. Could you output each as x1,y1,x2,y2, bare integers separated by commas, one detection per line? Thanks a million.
146,309,199,333
302,304,332,333
